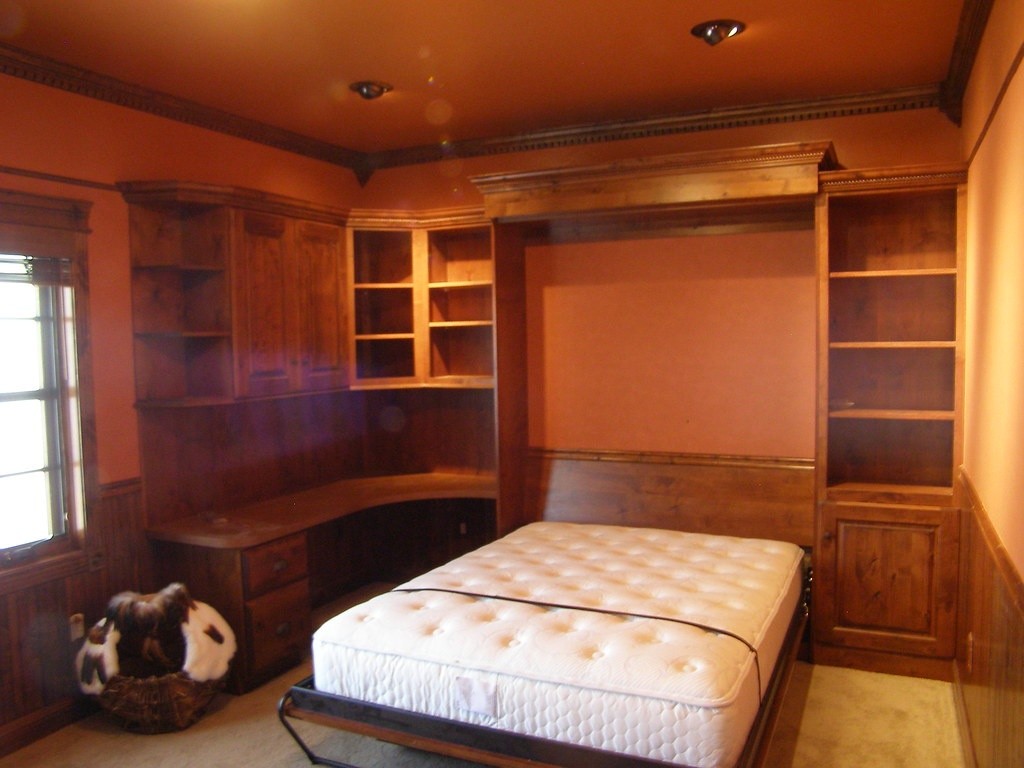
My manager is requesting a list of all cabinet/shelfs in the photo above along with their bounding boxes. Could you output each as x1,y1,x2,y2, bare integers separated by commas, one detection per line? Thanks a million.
117,177,495,408
813,183,964,658
145,469,499,695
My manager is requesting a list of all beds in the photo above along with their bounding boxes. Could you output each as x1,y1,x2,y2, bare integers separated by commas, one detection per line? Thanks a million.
280,520,817,768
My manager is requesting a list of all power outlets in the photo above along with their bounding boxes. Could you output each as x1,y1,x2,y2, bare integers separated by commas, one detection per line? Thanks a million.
69,613,85,641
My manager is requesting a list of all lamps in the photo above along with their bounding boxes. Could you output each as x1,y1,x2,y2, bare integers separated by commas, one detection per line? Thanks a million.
703,26,734,47
357,86,385,99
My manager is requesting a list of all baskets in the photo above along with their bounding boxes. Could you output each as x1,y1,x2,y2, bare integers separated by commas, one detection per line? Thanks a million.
75,584,235,734
